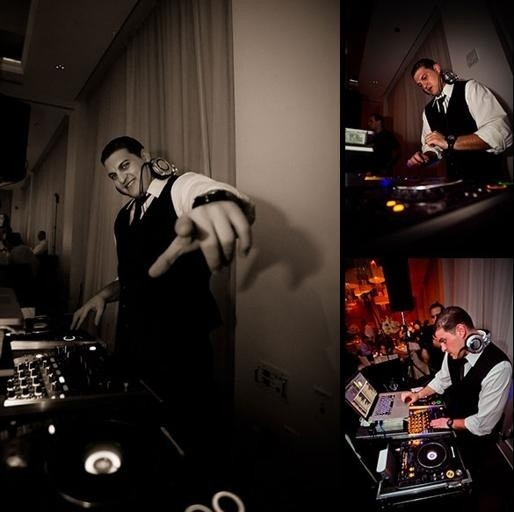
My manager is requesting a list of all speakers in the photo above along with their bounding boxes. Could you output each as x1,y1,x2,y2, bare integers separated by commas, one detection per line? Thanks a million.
382,264,415,311
0,94,32,189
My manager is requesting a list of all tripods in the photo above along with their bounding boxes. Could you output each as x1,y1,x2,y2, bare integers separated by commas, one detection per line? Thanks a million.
396,311,429,388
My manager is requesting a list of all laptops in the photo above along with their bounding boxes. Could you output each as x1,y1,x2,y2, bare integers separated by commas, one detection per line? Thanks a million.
343,370,411,421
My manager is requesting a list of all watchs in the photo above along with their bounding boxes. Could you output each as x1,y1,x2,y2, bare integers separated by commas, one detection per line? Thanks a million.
444,133,457,150
192,190,241,211
446,416,454,430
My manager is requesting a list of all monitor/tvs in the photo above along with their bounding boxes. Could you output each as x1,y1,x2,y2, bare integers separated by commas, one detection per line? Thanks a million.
344,126,368,147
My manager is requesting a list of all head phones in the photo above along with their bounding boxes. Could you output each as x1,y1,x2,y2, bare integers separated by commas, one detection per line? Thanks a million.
115,156,179,196
465,329,492,354
423,71,458,96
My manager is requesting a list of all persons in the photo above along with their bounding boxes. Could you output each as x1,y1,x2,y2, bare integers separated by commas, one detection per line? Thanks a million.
360,112,407,155
1,212,49,284
407,58,513,167
399,303,514,438
69,137,255,434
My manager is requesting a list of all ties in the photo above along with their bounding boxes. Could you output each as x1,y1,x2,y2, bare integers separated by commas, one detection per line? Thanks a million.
438,95,447,120
459,359,468,382
131,193,151,226
